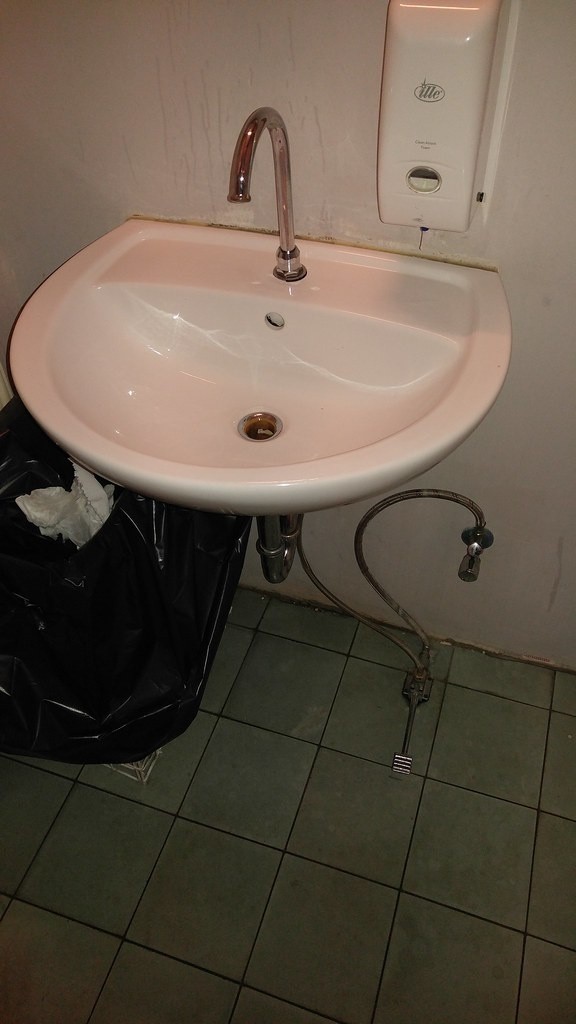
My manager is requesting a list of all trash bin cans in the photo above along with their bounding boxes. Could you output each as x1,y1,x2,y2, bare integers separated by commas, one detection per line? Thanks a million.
0,397,255,766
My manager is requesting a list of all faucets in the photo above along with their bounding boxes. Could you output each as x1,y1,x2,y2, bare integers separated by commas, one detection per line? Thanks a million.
226,106,305,281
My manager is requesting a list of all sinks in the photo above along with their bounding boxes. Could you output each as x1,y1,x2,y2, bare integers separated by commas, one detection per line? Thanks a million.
7,216,513,518
